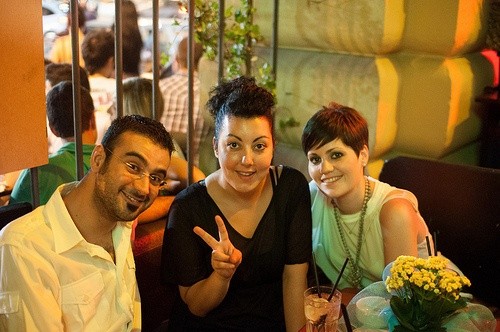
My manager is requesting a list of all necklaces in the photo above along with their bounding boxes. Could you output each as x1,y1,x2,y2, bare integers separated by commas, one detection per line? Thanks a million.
331,177,371,289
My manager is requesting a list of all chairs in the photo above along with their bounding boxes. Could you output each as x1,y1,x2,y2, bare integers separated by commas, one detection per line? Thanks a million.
378,154,500,310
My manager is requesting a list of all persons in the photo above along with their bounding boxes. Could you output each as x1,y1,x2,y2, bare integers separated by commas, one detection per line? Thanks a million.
8,0,206,208
302,101,472,291
159,76,312,332
0,114,175,332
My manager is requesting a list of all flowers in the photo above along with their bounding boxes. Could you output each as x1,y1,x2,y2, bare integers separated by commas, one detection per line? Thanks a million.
384,255,472,332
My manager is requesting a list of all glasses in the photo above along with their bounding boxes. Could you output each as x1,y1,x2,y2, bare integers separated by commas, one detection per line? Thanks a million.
103,144,168,187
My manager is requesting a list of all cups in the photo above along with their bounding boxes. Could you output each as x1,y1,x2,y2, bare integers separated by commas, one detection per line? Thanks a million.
304,286,342,332
